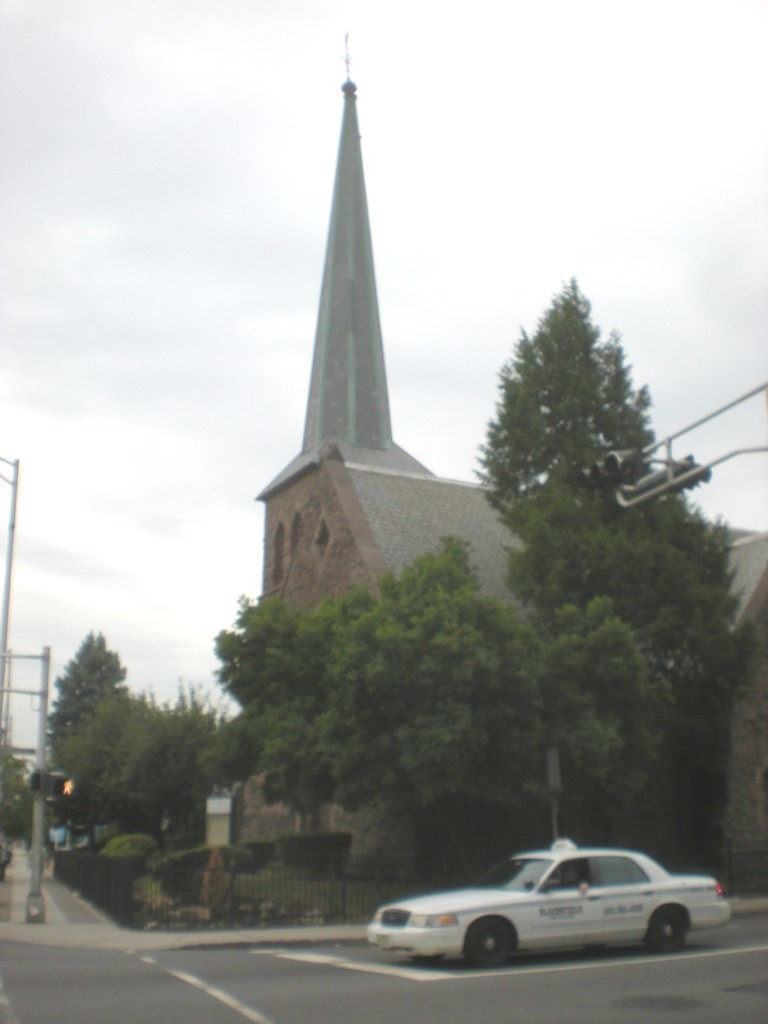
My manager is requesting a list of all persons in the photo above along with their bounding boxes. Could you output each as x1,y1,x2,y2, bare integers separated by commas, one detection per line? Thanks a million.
561,866,590,894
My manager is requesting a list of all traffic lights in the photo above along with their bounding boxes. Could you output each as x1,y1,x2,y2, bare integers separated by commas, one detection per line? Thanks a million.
635,458,709,505
30,771,45,791
50,774,74,797
574,448,644,493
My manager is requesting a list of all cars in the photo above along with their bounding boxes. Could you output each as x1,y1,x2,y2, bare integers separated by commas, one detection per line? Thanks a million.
366,837,732,967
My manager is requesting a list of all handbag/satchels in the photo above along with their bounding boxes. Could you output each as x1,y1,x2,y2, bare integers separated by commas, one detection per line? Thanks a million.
5,850,12,864
